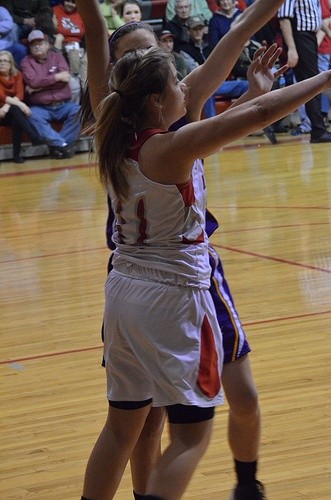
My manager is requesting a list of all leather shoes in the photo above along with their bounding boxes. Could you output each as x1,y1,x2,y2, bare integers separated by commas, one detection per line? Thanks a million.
310,131,331,143
264,126,277,144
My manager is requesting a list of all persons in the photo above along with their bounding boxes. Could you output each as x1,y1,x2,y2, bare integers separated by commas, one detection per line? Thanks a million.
82,42,331,500
72,0,292,500
0,0,331,164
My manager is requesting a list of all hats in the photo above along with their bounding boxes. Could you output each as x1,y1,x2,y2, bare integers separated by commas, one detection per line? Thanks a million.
157,30,177,40
27,29,46,44
188,16,205,29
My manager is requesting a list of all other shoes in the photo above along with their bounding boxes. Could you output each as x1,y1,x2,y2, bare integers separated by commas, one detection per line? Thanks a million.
13,154,25,163
49,146,74,159
32,137,46,146
